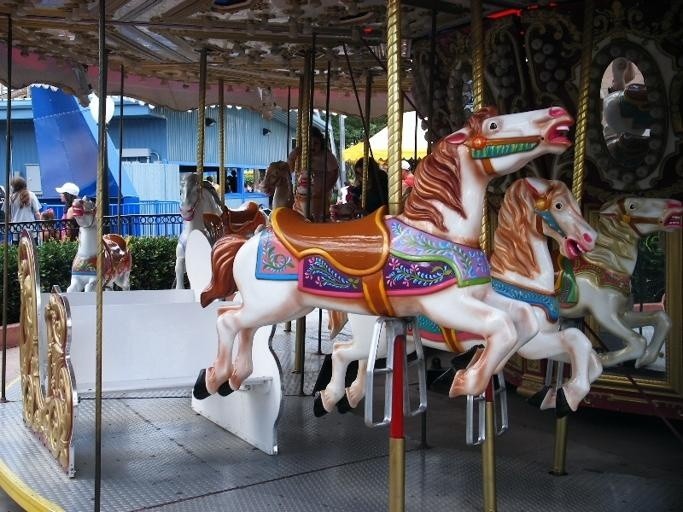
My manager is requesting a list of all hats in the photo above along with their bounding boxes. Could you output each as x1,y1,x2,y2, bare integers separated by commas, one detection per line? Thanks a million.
55,182,80,196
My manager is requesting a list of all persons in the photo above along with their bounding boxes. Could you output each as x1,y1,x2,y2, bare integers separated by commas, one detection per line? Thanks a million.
40,208,56,243
226,170,237,193
346,158,389,212
245,182,254,192
206,176,218,194
4,178,41,245
339,181,351,202
226,175,231,193
54,183,83,242
400,159,415,194
